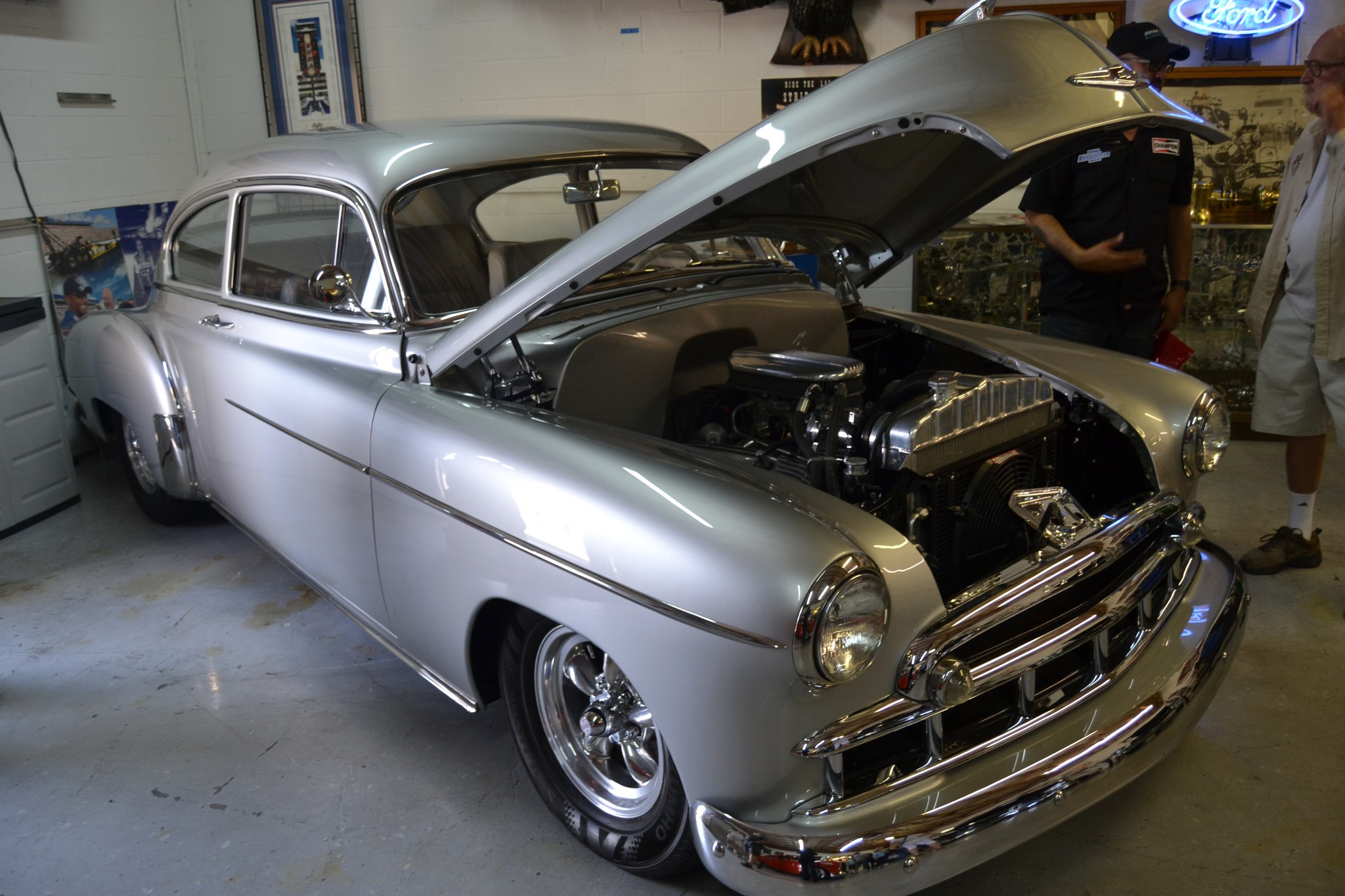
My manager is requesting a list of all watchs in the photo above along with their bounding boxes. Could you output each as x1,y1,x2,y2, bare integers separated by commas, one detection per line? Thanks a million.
1169,279,1191,291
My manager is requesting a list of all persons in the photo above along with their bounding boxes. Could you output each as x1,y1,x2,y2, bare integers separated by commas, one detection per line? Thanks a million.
1239,24,1344,575
61,274,92,344
131,236,156,307
1017,21,1195,360
96,287,120,310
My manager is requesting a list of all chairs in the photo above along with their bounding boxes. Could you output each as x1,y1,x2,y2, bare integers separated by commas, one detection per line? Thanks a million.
360,220,485,316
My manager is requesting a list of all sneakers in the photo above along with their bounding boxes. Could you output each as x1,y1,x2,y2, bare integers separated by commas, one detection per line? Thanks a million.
1238,527,1324,576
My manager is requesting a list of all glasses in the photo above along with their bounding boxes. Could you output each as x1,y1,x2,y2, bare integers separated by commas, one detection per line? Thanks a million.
1119,55,1177,75
1301,60,1345,78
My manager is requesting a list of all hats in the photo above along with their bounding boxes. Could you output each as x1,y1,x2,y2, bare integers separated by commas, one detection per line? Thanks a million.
1106,21,1190,63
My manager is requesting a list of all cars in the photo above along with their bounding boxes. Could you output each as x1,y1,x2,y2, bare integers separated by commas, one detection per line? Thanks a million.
65,0,1247,896
